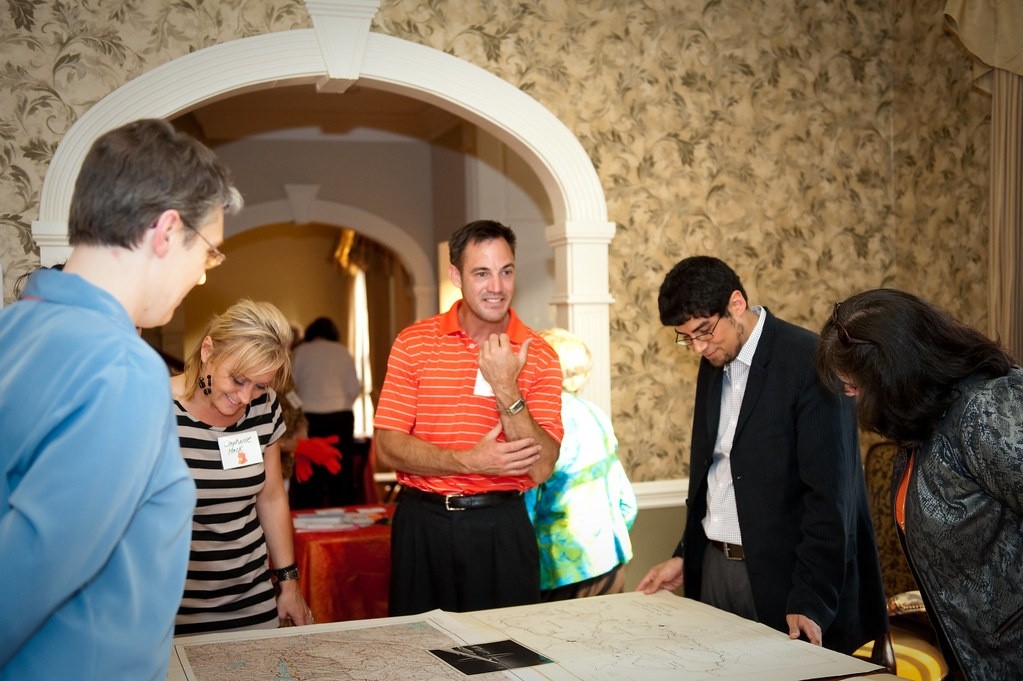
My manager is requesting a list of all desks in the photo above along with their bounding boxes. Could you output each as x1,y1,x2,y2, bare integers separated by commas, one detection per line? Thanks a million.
164,588,911,681
291,504,398,625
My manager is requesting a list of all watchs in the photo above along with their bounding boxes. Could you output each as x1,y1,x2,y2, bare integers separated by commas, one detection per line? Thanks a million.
276,568,299,582
495,394,526,415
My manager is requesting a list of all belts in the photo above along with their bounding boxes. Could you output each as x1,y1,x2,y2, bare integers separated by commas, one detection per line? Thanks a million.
400,483,522,512
709,540,744,560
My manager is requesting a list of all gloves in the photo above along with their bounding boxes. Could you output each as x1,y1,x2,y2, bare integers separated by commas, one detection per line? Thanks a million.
292,452,313,482
296,434,342,474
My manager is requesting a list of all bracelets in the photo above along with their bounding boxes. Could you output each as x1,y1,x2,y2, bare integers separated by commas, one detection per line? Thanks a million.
273,560,297,575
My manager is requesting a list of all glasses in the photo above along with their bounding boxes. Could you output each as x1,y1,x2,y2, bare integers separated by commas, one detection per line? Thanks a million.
831,301,876,346
149,209,225,268
675,318,721,345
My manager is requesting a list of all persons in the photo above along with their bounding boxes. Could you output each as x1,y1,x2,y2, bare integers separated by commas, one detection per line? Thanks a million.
634,256,877,658
815,288,1023,681
522,328,638,606
0,118,235,681
169,295,317,639
372,219,564,618
289,316,361,513
